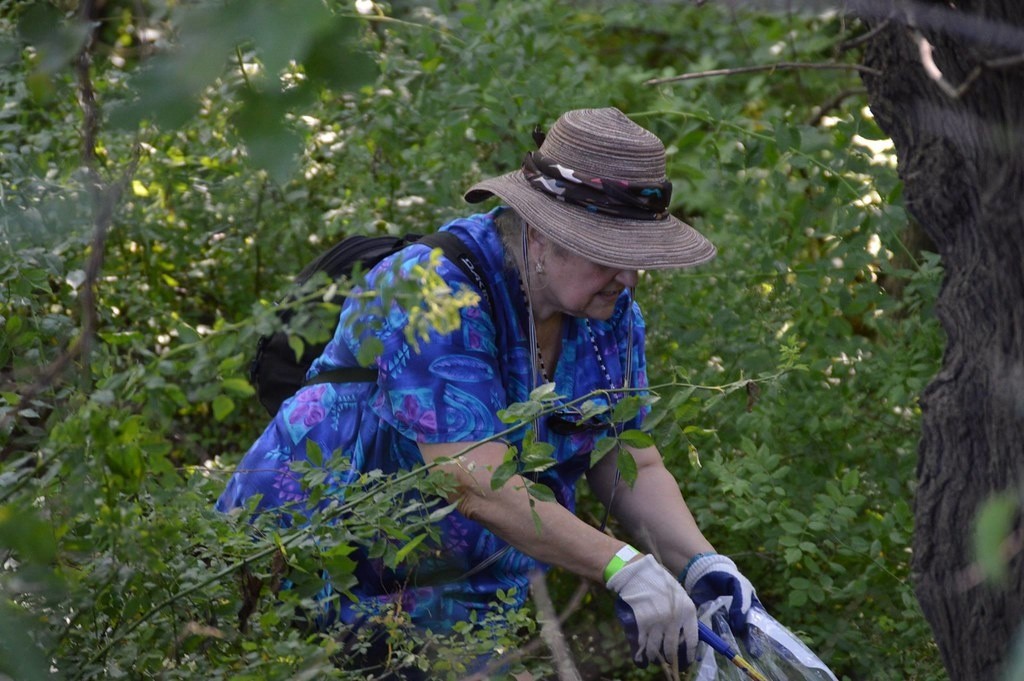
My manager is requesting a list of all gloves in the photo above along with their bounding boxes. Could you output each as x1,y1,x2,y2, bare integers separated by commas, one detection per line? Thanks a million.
604,553,699,673
676,554,796,670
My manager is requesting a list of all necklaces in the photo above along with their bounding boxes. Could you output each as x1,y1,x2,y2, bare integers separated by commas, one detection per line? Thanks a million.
514,259,620,426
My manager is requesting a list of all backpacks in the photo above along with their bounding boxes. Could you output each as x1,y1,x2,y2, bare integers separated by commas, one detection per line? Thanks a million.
244,231,469,417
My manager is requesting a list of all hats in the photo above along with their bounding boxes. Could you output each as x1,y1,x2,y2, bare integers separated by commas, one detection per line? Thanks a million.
464,106,718,271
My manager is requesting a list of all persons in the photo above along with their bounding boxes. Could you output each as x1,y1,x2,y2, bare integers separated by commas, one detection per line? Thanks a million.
210,103,764,680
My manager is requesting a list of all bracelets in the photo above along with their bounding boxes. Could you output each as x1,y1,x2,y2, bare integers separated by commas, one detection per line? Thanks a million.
603,544,637,586
